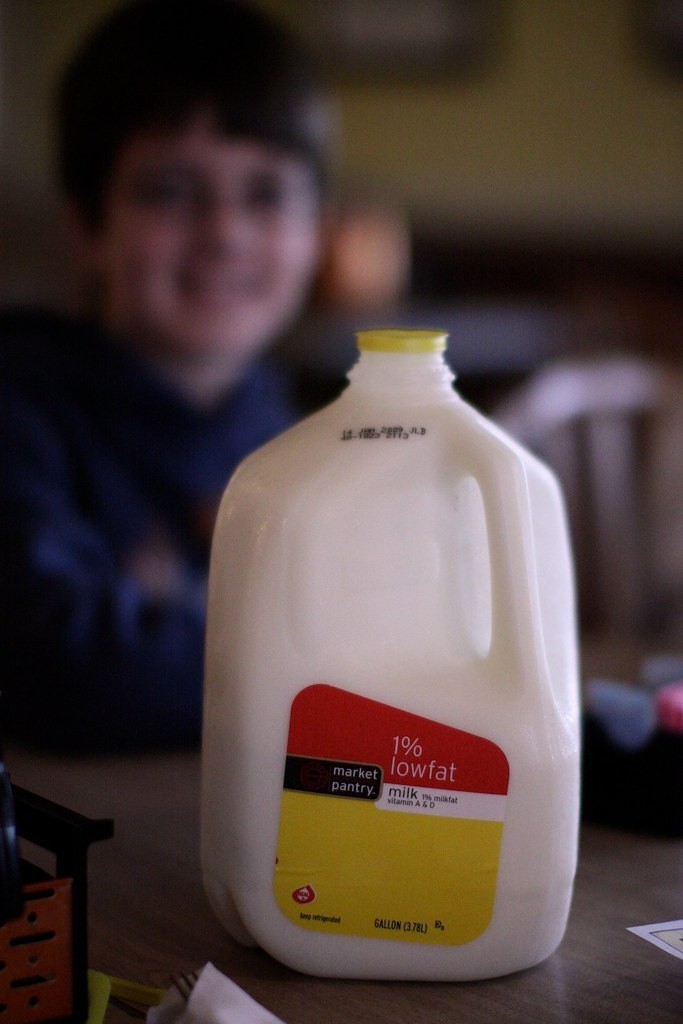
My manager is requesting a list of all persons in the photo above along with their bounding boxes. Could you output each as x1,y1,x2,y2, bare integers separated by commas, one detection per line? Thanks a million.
0,0,336,754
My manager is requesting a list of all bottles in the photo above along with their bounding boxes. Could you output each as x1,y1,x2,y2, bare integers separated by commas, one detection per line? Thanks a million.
204,331,582,983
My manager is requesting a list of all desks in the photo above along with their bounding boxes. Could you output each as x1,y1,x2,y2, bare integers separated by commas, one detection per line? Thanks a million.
0,639,683,1024
